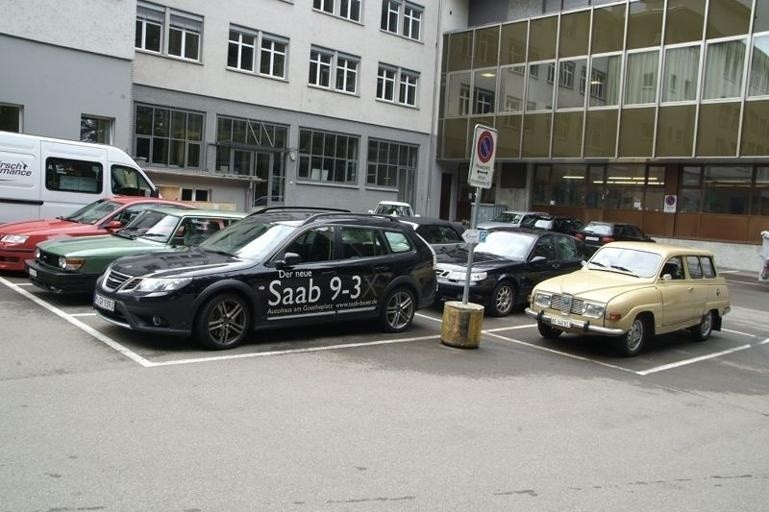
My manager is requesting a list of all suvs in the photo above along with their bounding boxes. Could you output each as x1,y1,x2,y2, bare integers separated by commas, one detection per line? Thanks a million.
525,240,731,358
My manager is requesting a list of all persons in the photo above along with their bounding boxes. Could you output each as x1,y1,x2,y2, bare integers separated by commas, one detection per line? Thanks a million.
183,221,220,245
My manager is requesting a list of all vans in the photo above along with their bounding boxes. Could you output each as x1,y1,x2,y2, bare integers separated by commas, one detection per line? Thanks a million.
0,130,165,227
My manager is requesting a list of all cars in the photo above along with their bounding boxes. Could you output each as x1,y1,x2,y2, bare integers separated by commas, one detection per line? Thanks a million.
433,227,595,317
477,211,552,231
255,196,283,206
367,200,421,220
571,220,657,259
24,208,254,297
91,205,439,351
384,216,466,251
522,214,586,235
0,194,227,275
